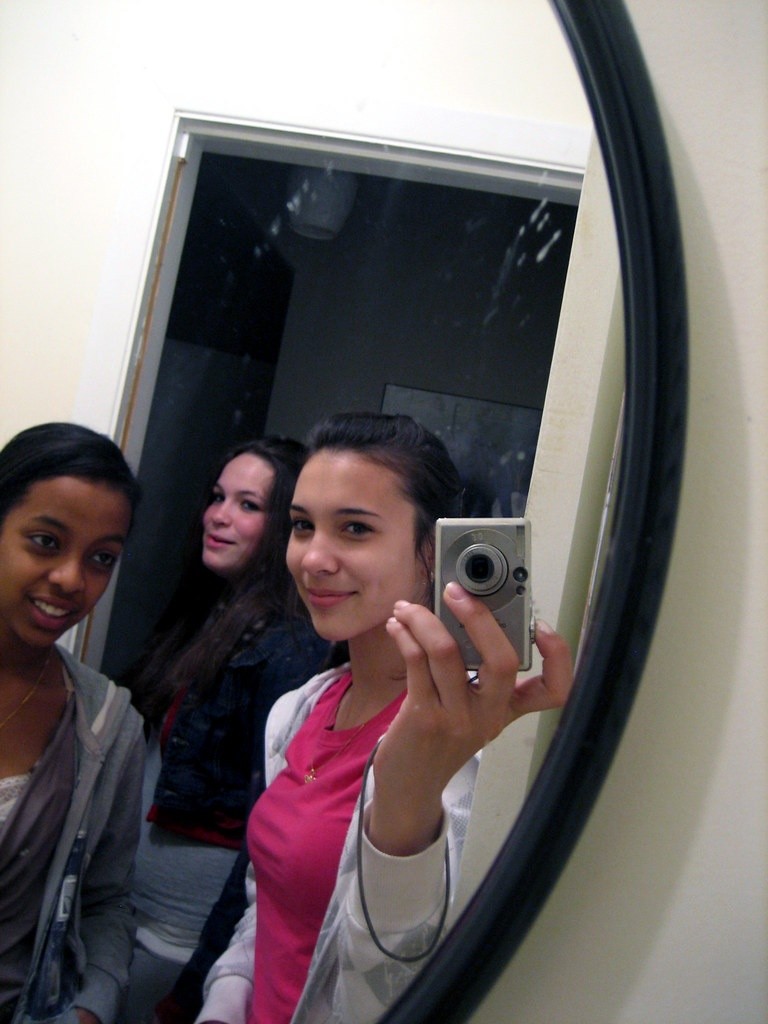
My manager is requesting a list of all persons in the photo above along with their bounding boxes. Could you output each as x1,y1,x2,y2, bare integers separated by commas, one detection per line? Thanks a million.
196,413,571,1024
0,423,148,1024
147,435,350,1024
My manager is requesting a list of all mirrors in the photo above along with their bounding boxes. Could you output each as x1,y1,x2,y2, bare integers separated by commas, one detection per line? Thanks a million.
0,1,688,1024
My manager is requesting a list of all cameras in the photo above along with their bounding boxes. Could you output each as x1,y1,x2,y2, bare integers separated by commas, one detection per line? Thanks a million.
433,517,538,671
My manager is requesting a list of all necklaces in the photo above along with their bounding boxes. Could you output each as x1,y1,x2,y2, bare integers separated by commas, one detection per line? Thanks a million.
0,646,55,727
305,683,365,784
